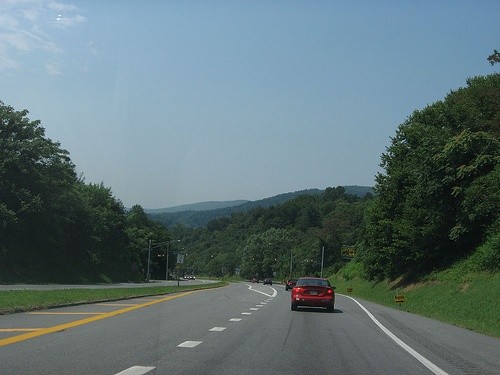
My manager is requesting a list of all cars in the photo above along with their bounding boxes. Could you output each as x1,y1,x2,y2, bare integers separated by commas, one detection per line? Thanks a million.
184,275,196,280
252,278,260,283
289,277,337,312
263,278,272,285
285,278,299,291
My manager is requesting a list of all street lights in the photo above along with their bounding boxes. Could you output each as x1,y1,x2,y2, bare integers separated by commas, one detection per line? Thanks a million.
166,246,185,281
268,242,294,278
147,239,181,281
